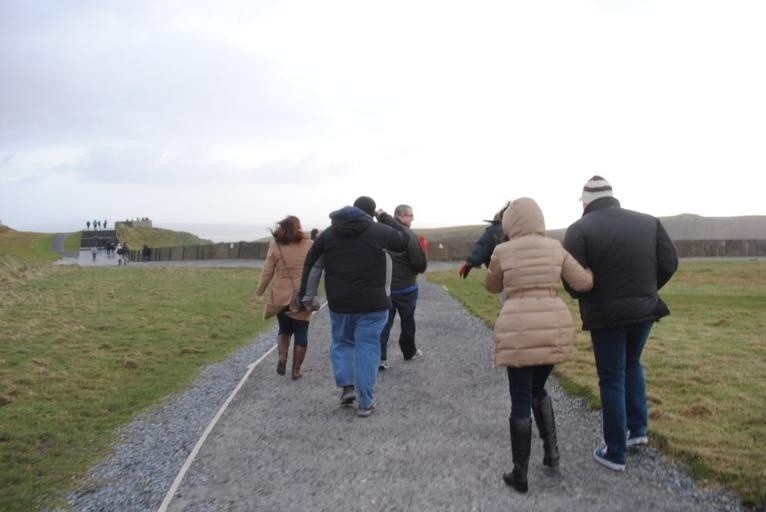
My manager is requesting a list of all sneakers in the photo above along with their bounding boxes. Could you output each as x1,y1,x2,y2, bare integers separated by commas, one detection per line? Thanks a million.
625,431,647,446
357,402,376,415
340,386,355,404
593,445,625,471
410,349,422,360
378,360,389,369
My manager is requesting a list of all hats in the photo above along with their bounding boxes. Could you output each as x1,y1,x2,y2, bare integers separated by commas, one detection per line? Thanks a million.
581,176,611,204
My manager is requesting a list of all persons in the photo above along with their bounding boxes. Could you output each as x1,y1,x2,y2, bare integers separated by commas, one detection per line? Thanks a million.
141,244,150,261
561,175,677,472
299,196,427,416
481,197,595,493
90,241,129,266
85,219,107,229
460,201,510,279
256,216,314,379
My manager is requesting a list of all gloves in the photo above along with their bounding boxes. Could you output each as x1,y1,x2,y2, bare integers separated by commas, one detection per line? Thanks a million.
459,263,470,278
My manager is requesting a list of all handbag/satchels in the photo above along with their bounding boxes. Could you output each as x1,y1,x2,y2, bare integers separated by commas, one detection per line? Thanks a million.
293,288,319,313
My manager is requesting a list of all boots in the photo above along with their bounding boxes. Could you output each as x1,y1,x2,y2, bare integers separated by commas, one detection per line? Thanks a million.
531,396,559,467
292,345,305,378
502,418,531,492
276,335,290,375
